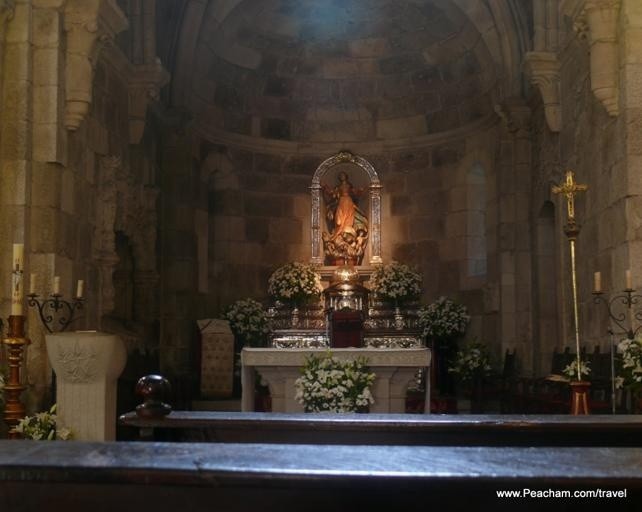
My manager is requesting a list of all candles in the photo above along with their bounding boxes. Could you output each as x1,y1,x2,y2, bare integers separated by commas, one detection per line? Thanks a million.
11,243,23,317
29,273,84,297
593,269,633,292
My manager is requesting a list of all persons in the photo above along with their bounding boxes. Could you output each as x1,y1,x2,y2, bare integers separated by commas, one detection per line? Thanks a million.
321,171,372,261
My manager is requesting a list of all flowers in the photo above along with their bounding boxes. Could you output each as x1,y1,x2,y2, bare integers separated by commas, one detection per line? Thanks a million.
560,356,592,381
363,259,491,382
293,350,377,413
613,339,642,389
225,261,324,338
7,402,77,442
